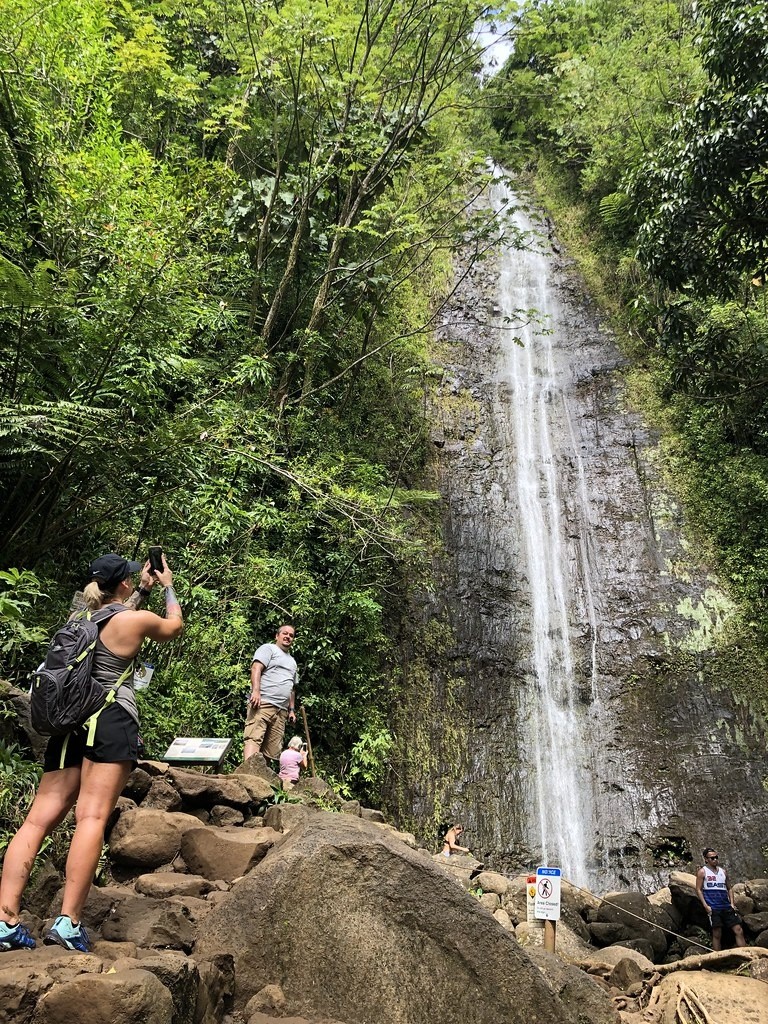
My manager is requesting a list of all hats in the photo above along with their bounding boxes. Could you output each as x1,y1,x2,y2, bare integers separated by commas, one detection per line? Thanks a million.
703,848,715,864
91,553,142,594
83,581,106,609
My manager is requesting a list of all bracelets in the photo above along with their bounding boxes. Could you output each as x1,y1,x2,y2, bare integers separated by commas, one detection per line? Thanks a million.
289,708,295,711
135,587,150,598
159,586,175,594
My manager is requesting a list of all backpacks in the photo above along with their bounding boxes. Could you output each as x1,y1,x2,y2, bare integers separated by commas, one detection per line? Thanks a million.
30,603,136,735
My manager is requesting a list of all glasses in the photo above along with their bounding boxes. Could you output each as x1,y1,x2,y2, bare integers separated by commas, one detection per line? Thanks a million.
707,855,719,860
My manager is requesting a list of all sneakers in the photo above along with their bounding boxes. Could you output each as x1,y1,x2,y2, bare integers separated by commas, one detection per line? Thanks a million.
0,921,36,952
44,915,89,952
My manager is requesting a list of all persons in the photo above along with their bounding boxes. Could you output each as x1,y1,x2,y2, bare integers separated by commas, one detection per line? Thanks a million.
440,824,470,857
243,624,298,765
0,553,184,952
279,735,308,784
696,848,746,951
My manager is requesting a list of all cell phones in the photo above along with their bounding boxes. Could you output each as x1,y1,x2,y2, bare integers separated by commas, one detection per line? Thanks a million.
303,743,307,752
148,546,165,575
